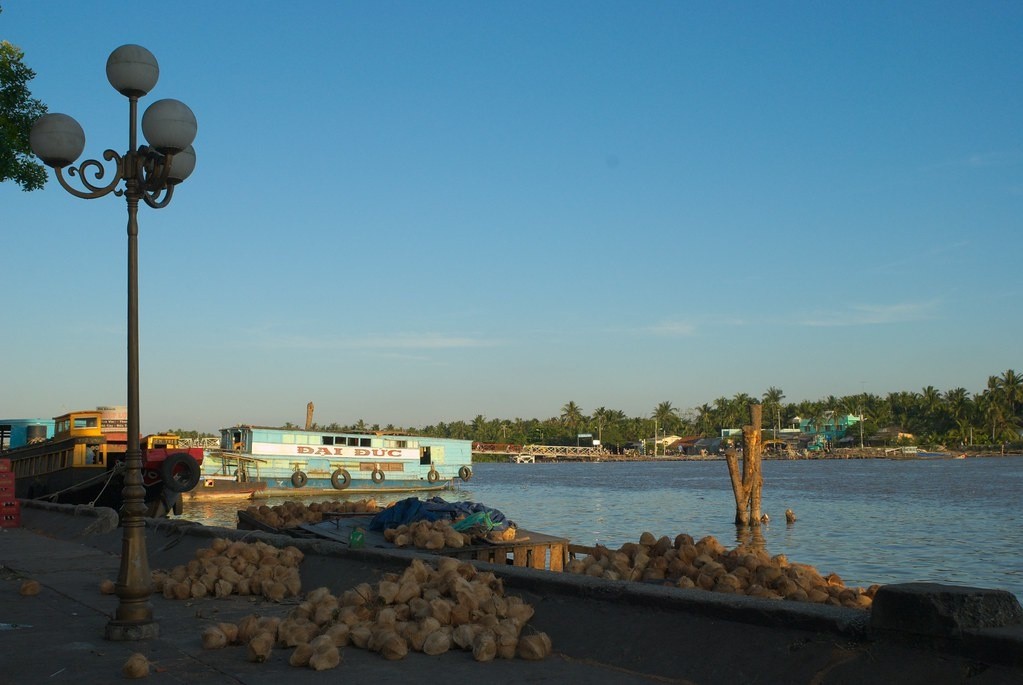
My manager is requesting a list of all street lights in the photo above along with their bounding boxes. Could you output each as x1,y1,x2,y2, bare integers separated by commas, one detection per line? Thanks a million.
30,43,199,600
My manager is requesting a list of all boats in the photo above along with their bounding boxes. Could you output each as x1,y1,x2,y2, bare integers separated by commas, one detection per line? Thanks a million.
0,403,204,520
183,425,471,503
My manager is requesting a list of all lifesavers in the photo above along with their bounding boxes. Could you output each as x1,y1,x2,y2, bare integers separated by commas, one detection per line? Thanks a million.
234,442,246,450
